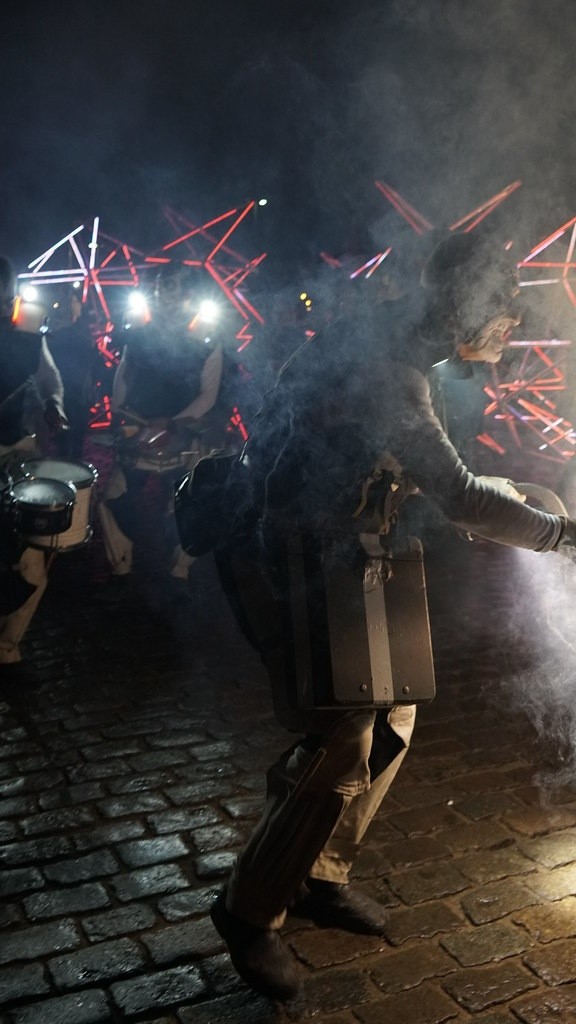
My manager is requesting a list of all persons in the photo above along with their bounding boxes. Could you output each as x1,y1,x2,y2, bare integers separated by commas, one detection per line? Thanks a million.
1,229,576,996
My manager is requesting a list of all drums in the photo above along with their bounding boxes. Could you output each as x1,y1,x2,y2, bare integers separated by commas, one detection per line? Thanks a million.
8,476,77,537
128,425,194,473
17,454,99,554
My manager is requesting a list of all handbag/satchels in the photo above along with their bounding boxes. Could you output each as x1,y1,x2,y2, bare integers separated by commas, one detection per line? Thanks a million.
173,446,251,558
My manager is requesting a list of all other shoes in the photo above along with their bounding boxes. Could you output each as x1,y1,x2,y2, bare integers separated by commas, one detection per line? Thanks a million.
0,661,37,680
289,874,391,936
211,885,301,1000
110,574,128,586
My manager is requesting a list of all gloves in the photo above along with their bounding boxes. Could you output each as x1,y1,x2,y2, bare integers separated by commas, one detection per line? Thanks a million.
552,514,576,563
